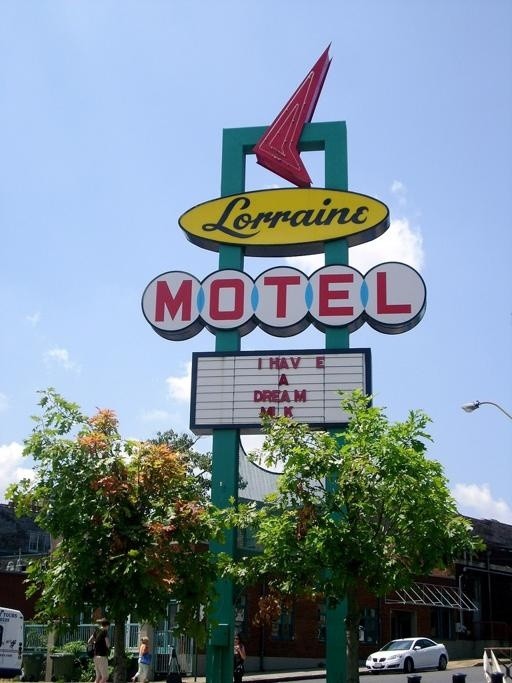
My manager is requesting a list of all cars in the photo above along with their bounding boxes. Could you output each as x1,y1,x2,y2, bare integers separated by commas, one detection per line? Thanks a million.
365,636,449,675
344,618,374,643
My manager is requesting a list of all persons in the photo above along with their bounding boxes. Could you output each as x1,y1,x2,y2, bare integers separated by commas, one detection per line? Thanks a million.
88,618,114,683
131,636,154,683
234,634,247,682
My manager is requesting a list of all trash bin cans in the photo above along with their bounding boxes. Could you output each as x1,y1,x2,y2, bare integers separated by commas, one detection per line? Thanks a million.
21,653,44,682
49,652,75,683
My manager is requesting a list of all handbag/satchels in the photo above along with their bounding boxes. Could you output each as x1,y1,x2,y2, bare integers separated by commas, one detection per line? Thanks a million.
139,654,152,666
86,644,95,658
235,663,245,677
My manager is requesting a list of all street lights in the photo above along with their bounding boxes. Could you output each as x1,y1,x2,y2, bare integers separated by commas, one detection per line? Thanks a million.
460,400,511,420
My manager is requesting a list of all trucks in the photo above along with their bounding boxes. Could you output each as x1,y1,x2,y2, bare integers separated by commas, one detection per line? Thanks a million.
1,607,24,679
